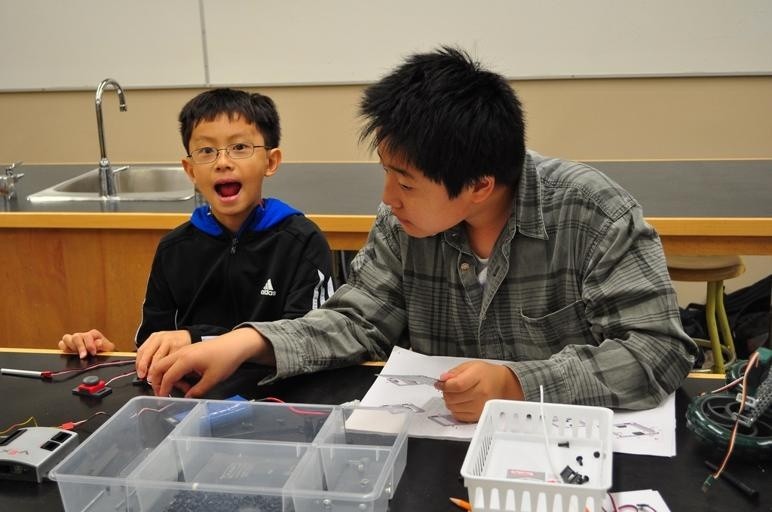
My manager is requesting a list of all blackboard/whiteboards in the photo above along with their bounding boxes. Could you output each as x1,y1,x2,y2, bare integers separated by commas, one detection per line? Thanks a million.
0,0,772,94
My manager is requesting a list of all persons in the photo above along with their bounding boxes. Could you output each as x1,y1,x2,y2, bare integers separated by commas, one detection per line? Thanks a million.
52,87,333,361
144,45,700,425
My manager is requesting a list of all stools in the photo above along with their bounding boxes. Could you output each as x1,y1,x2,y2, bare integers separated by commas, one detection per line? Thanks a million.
666,255,745,373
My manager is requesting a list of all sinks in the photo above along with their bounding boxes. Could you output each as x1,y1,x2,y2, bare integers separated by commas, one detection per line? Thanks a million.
26,165,197,202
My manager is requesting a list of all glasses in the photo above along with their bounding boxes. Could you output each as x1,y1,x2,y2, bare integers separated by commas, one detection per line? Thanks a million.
186,141,274,166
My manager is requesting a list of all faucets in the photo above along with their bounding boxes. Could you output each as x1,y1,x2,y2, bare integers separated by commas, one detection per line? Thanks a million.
93,76,129,196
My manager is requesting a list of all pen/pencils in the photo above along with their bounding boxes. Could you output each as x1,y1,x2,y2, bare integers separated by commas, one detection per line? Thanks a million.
449,497,471,510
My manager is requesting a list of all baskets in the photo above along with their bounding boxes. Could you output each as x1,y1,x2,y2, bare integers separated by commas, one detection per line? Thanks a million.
459,397,616,511
